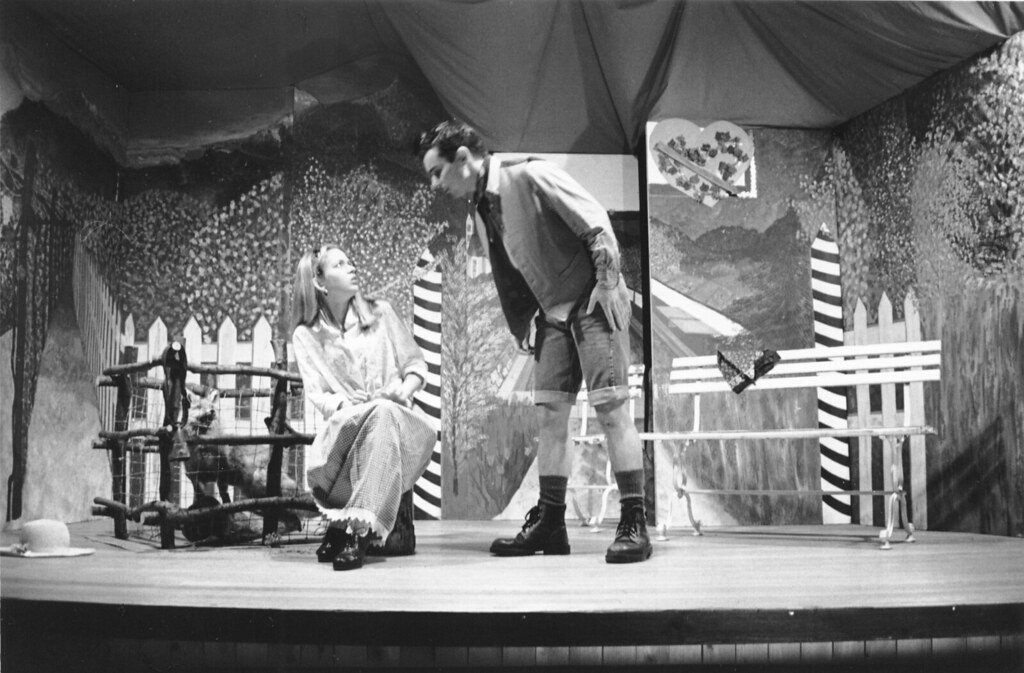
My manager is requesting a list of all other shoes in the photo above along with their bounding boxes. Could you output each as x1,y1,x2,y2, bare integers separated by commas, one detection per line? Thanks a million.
333,527,373,570
315,526,347,562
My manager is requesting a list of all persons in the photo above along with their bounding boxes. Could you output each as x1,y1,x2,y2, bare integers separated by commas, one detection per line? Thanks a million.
292,244,437,570
418,119,653,563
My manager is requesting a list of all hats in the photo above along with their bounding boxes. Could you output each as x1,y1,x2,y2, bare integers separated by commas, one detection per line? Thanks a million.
0,519,95,558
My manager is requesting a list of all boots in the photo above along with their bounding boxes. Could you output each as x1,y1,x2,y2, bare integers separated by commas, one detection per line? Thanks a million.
604,499,653,563
489,499,571,555
